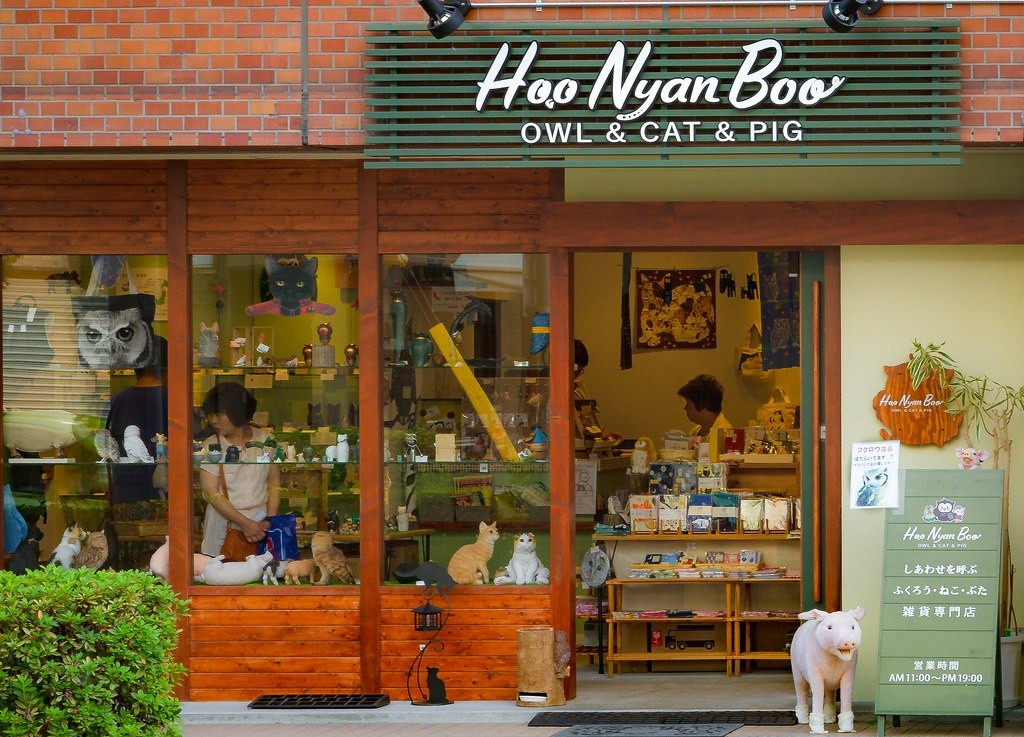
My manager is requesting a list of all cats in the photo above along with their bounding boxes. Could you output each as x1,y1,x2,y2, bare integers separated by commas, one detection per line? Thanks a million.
492,531,549,585
8,522,87,575
243,254,337,316
447,521,500,585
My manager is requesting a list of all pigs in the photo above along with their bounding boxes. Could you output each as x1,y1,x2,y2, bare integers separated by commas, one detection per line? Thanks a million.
261,558,319,585
148,533,274,586
789,607,866,733
2,408,98,459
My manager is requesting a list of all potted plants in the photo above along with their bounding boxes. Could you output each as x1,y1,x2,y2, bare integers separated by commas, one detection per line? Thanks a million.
111,500,200,536
907,338,1024,709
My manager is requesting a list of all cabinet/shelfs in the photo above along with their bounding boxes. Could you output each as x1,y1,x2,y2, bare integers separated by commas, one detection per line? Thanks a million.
0,233,572,596
576,572,617,674
592,463,801,677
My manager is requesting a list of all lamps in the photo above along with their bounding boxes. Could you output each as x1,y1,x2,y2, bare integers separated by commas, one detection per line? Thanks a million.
822,0,883,33
415,0,471,40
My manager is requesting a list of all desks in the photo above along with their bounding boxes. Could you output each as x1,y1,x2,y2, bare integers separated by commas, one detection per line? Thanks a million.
117,528,436,581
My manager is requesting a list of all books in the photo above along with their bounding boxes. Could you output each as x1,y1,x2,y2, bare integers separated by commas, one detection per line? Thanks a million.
627,494,801,579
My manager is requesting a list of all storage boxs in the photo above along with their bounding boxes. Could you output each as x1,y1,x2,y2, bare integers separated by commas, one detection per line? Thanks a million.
299,541,419,586
418,493,454,521
743,454,794,463
529,506,549,521
455,506,493,521
620,623,648,673
652,621,727,671
744,454,793,463
584,619,617,647
628,492,802,534
710,428,724,463
628,562,765,571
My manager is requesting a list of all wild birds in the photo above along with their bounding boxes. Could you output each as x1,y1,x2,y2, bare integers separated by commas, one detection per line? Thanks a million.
70,529,109,573
122,424,153,464
93,429,121,465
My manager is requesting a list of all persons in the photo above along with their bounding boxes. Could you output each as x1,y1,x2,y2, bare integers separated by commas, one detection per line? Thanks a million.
4,406,47,576
104,334,205,502
677,373,732,444
200,382,280,563
573,337,624,445
